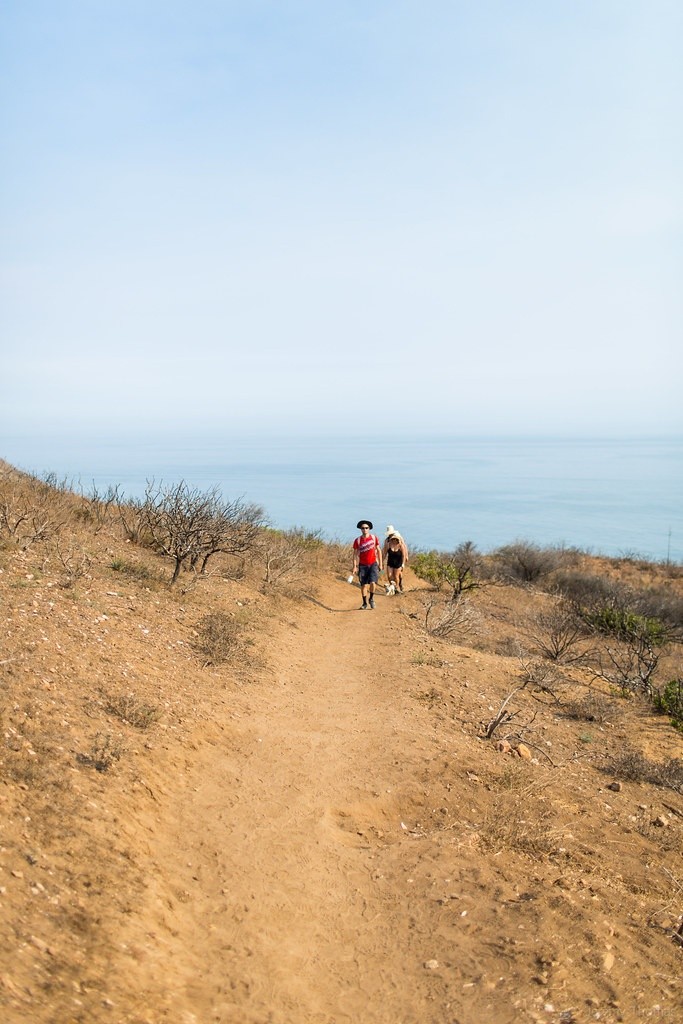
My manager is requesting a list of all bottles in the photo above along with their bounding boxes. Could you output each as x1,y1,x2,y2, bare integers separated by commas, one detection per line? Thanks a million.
347,573,354,584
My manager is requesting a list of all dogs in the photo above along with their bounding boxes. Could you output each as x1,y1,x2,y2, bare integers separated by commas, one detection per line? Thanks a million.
383,581,396,595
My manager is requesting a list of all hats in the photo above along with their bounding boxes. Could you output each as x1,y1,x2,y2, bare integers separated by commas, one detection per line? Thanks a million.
357,520,373,530
384,525,398,535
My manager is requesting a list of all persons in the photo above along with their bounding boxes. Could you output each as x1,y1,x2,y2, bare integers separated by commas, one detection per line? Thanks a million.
351,519,383,609
381,534,405,593
379,525,409,592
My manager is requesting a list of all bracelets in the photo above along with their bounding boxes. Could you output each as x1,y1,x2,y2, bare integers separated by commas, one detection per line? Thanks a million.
401,563,404,567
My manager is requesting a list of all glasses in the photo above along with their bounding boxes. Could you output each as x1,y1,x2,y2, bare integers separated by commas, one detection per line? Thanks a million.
361,527,369,529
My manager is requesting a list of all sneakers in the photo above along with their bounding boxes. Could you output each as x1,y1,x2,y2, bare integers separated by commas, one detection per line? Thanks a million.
360,603,368,609
369,599,375,609
